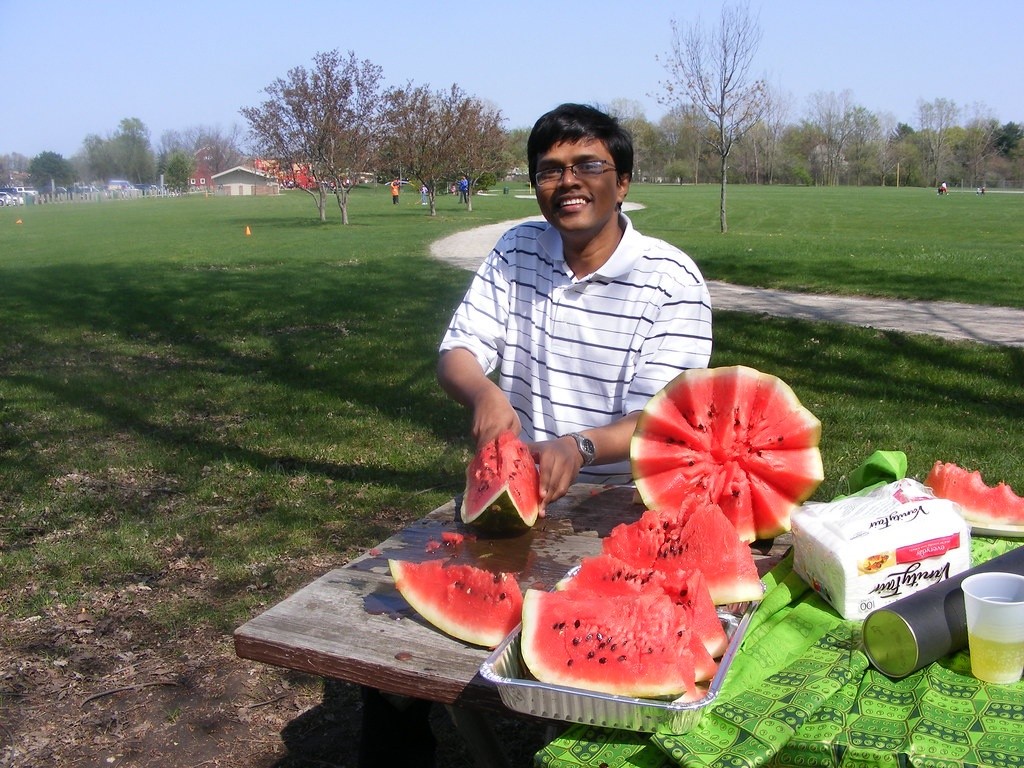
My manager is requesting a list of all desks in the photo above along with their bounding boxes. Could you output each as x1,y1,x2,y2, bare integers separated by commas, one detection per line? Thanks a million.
232,481,829,768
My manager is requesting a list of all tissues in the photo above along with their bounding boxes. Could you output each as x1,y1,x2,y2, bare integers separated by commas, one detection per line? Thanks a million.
789,478,968,622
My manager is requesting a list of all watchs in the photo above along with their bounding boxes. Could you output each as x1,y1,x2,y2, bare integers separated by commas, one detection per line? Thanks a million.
562,432,597,468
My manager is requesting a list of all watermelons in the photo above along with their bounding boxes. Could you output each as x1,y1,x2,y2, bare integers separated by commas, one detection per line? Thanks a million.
519,495,765,702
922,460,1024,527
630,362,826,545
460,431,541,532
388,557,522,647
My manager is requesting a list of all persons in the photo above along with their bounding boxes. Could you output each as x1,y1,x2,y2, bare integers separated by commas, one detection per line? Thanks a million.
455,175,469,204
420,183,429,205
938,180,986,196
450,183,456,195
390,177,401,205
436,103,713,517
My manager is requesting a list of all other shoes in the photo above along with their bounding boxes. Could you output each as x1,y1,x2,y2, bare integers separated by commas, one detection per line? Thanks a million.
465,201,468,204
421,202,427,205
458,202,463,204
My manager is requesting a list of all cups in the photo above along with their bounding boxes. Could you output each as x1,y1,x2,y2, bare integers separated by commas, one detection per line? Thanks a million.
961,571,1024,683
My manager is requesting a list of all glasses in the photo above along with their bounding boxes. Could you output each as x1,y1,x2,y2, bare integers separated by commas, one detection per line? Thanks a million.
535,160,617,187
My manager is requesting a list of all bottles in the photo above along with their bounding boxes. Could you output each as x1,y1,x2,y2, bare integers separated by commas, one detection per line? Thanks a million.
862,545,1024,678
